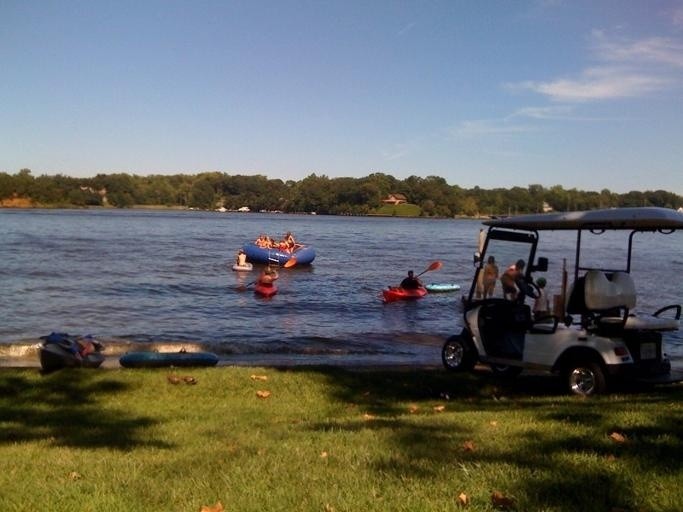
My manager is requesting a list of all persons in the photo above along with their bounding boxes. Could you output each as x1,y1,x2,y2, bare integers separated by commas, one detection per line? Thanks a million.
400,270,422,289
500,259,526,299
236,249,247,266
259,267,279,286
532,277,550,319
479,229,485,253
476,256,499,298
254,231,296,254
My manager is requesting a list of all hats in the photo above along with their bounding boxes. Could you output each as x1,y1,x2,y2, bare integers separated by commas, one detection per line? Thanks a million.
536,277,546,287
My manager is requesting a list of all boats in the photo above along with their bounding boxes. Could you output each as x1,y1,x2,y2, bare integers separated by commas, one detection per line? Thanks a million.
233,262,253,271
255,272,278,297
119,349,220,367
383,283,461,305
241,238,315,265
40,344,104,371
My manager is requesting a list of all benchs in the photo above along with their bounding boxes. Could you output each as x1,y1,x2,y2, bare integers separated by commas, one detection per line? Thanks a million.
532,267,680,338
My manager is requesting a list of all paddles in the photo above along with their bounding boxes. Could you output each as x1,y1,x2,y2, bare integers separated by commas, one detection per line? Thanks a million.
235,257,297,293
415,261,440,277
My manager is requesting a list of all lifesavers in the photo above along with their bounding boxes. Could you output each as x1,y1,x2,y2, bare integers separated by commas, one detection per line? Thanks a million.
120,350,219,368
